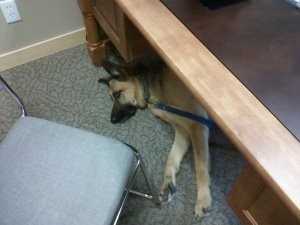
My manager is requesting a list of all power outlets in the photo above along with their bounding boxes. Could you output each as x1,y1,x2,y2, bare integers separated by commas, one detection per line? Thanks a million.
0,0,22,24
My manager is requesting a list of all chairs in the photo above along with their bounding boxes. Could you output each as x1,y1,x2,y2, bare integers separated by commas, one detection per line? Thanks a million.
0,74,162,225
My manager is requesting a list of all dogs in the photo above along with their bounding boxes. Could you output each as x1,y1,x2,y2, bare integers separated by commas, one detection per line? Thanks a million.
98,58,239,222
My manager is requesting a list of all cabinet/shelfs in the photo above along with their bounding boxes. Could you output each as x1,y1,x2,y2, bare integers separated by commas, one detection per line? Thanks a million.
91,0,155,64
225,163,300,225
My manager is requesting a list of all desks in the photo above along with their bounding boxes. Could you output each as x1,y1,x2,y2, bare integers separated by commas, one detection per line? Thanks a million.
75,0,300,225
